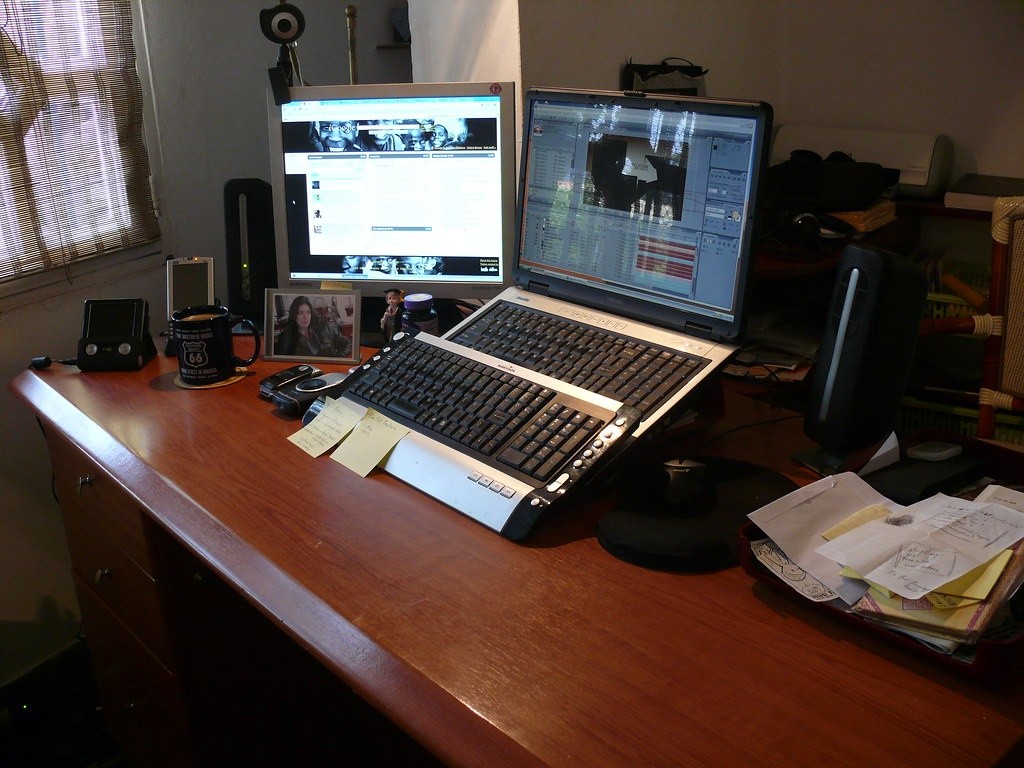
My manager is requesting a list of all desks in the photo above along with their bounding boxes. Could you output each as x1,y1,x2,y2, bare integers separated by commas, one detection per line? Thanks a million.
8,336,1024,768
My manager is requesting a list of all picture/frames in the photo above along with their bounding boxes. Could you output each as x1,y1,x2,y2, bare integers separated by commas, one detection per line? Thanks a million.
263,287,362,365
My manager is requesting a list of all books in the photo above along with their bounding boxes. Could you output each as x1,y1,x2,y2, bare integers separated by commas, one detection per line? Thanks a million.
719,325,820,385
855,536,1024,643
830,200,896,233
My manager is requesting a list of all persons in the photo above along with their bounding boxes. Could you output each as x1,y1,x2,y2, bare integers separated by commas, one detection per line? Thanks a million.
274,296,354,359
307,120,460,276
381,288,404,343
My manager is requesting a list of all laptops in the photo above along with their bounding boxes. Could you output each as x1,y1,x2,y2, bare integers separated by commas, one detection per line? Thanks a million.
442,85,774,483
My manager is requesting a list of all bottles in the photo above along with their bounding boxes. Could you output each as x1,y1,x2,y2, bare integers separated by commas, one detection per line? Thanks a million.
402,293,439,336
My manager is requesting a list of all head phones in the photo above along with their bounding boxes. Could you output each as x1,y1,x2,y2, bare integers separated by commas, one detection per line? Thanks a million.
774,203,857,252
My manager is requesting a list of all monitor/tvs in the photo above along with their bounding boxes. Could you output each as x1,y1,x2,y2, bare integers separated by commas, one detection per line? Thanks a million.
268,82,516,348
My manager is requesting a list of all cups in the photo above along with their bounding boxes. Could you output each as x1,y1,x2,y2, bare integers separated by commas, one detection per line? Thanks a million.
169,307,261,386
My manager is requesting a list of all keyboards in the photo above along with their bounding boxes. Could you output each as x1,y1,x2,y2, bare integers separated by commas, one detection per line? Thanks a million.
301,326,643,540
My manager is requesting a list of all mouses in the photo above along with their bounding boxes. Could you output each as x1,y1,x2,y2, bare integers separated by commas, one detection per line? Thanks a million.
653,456,715,516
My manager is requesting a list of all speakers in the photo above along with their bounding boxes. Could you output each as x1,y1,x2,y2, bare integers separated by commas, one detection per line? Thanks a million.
791,245,924,475
223,178,278,335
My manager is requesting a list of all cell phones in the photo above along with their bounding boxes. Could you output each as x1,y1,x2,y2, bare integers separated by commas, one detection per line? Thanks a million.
259,365,312,397
